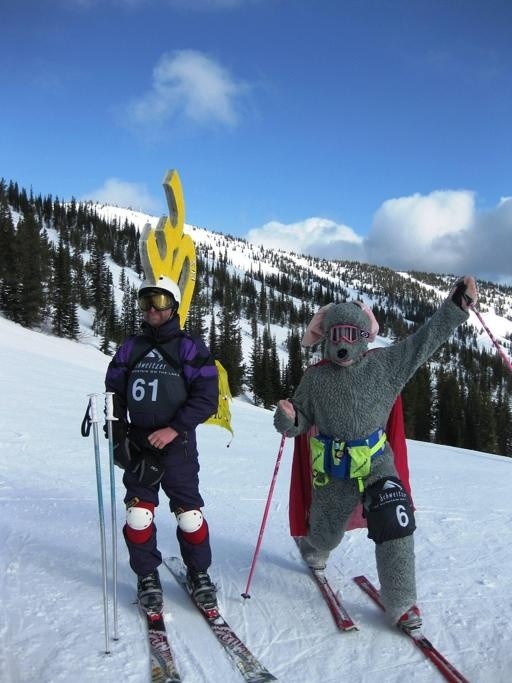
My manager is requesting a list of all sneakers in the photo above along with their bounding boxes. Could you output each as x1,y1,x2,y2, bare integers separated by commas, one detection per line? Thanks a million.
186,566,217,608
308,557,327,572
137,567,163,608
400,605,422,631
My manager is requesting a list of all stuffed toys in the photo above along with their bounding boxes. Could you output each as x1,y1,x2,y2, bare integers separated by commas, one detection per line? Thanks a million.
273,275,477,631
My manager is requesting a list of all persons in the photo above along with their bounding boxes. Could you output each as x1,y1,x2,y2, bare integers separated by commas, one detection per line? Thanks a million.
102,272,220,616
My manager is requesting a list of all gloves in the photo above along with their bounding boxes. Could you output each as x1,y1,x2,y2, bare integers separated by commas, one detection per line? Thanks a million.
103,418,166,487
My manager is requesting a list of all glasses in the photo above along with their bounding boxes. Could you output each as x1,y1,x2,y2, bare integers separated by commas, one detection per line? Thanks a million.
324,325,370,344
138,292,176,311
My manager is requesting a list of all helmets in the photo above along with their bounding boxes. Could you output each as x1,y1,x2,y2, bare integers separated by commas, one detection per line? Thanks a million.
139,275,182,312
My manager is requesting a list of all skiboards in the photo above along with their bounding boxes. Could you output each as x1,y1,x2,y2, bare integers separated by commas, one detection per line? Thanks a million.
147,557,278,683
294,536,469,683
